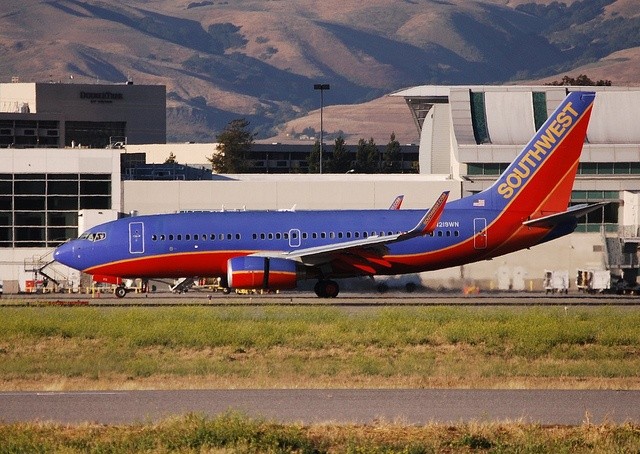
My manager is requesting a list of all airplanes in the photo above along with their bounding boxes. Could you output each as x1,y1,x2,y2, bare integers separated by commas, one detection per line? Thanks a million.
54,92,611,298
